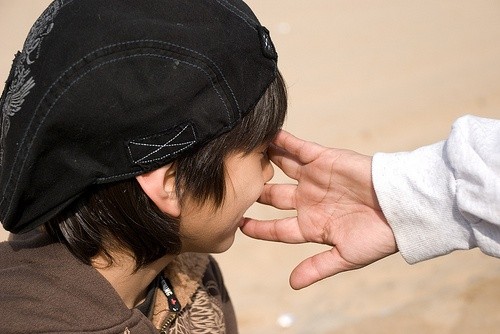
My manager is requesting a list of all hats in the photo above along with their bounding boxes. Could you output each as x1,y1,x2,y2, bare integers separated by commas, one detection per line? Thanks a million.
0,0,279,232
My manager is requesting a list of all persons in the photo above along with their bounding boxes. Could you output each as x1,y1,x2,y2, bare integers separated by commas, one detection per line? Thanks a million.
240,115,500,289
0,0,287,334
